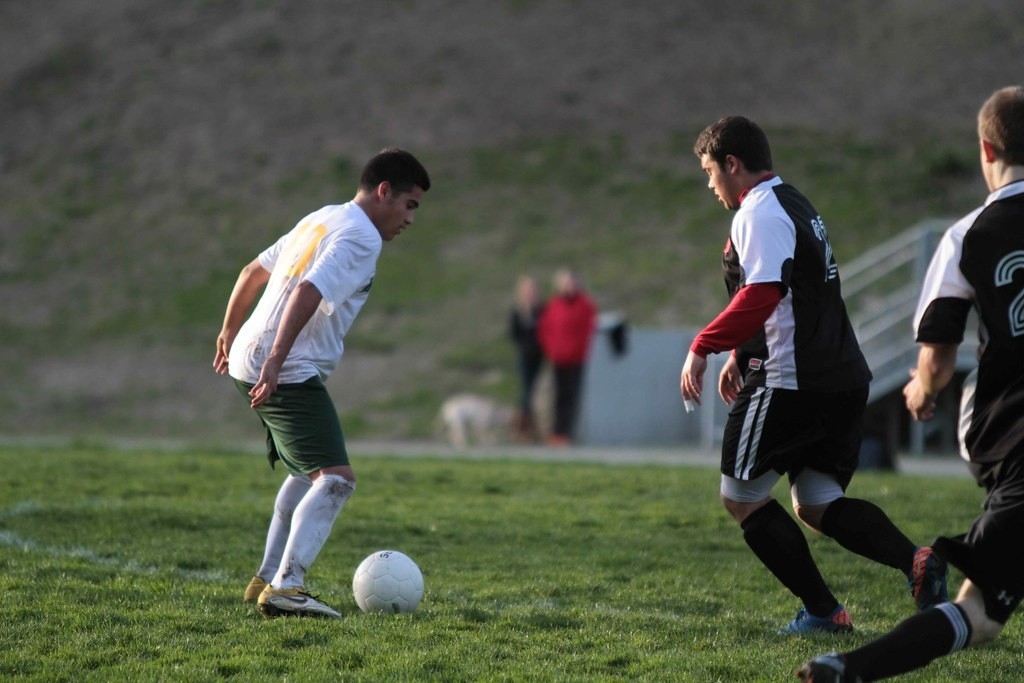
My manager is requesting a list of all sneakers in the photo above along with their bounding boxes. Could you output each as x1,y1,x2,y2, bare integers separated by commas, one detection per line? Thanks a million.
257,583,343,618
797,651,861,683
775,602,853,635
243,576,268,602
909,545,949,613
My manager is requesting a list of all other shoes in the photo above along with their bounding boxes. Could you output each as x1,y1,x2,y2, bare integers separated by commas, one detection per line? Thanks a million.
544,433,570,446
513,413,533,442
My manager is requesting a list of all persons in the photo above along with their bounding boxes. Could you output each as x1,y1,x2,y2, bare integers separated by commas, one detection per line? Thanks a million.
679,115,948,634
795,85,1024,683
509,277,546,438
537,271,596,446
212,147,430,619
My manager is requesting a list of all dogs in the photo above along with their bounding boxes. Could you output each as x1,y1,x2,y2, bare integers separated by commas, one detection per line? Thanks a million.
434,393,521,454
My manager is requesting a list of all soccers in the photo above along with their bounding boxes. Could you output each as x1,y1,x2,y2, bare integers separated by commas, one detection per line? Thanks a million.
352,548,425,616
